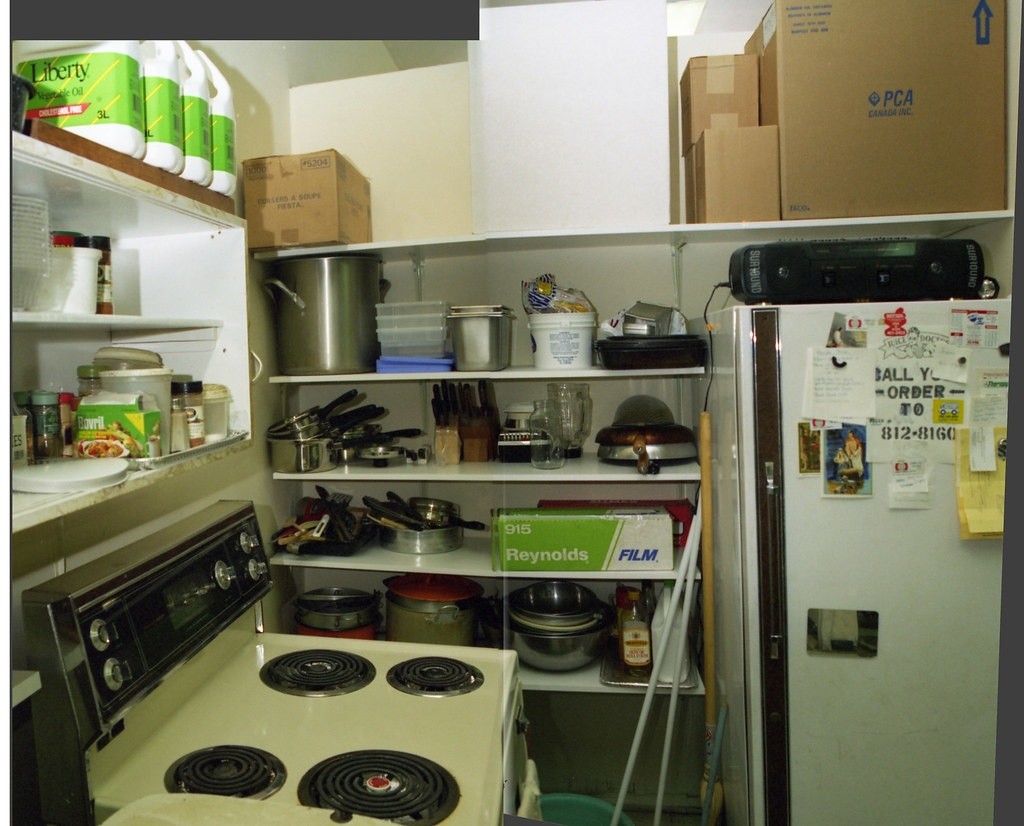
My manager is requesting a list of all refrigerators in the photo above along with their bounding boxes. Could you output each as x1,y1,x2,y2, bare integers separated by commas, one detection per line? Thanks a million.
685,297,1013,826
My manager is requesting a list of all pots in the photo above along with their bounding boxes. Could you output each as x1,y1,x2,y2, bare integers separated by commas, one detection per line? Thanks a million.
266,388,422,474
293,585,384,640
383,589,481,647
595,393,698,475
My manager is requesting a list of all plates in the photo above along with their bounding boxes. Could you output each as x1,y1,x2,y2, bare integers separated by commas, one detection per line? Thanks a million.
11,456,129,493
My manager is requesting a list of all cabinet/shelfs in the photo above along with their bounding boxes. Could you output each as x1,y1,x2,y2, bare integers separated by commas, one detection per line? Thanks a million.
10,128,249,535
261,211,1017,696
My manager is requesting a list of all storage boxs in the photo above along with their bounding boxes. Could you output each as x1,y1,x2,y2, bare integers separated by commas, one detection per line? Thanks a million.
243,150,375,252
483,500,693,574
681,0,1007,226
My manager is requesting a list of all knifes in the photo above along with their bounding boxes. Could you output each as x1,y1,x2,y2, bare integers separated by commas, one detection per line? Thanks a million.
431,379,490,426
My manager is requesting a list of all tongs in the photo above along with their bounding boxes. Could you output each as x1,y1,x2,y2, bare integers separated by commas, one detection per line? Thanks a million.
362,491,430,528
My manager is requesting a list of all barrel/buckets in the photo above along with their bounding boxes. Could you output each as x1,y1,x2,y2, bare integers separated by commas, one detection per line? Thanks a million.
100,368,172,459
260,256,382,375
526,313,596,368
12,40,237,197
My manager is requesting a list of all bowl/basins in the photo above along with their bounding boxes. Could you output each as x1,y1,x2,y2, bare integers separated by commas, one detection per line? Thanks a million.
481,581,613,672
54,247,103,312
12,195,52,313
537,793,635,826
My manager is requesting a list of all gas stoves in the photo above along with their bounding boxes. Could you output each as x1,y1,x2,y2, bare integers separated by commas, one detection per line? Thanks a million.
21,498,524,826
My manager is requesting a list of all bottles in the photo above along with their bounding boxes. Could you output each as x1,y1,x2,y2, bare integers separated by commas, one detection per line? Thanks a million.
528,400,565,470
615,581,675,683
546,383,593,458
12,346,229,459
